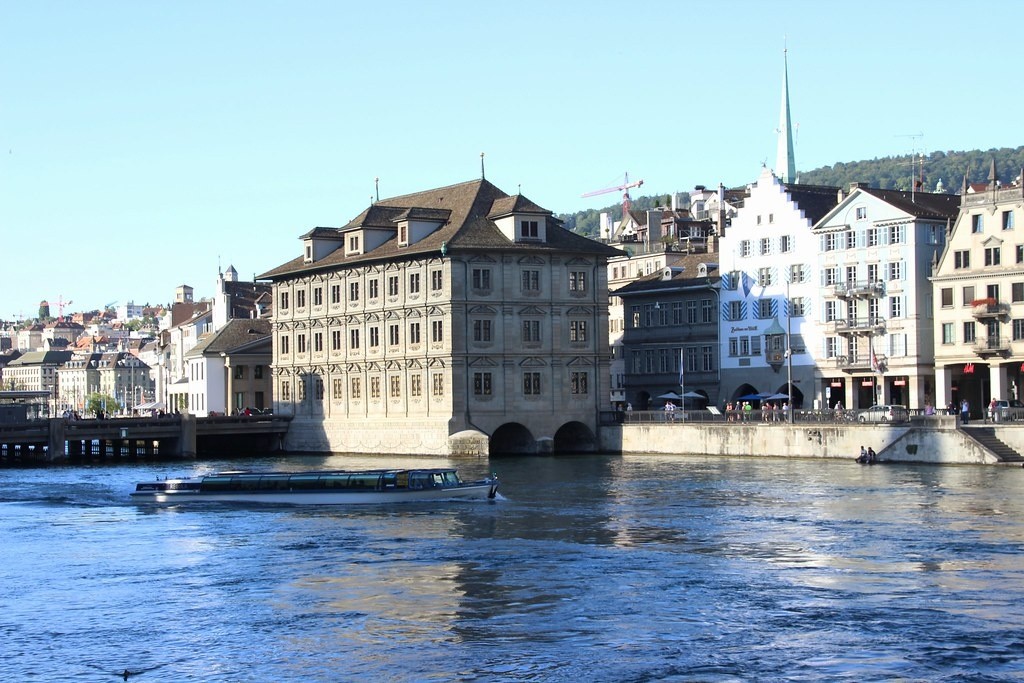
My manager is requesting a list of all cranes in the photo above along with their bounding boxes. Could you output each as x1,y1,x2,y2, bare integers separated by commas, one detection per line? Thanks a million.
577,170,644,219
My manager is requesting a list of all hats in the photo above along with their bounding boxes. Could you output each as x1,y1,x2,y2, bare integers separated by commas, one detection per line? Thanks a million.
737,402,740,404
746,402,749,404
742,402,746,404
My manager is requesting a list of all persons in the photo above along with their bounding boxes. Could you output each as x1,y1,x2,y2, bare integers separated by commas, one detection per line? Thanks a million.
959,398,998,425
617,400,678,426
924,401,933,418
835,402,847,424
719,400,790,427
60,406,255,422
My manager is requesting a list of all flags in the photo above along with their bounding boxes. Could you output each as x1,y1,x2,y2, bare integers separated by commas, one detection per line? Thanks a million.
870,347,885,374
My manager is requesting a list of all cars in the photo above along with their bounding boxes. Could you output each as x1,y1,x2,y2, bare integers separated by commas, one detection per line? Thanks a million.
240,407,263,416
988,399,1024,421
858,404,907,424
262,408,273,416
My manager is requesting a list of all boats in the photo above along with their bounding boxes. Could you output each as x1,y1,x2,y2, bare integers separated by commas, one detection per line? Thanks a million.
128,460,503,501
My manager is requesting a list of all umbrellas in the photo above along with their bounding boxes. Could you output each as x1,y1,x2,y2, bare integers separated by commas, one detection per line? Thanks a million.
654,390,706,403
736,391,794,400
